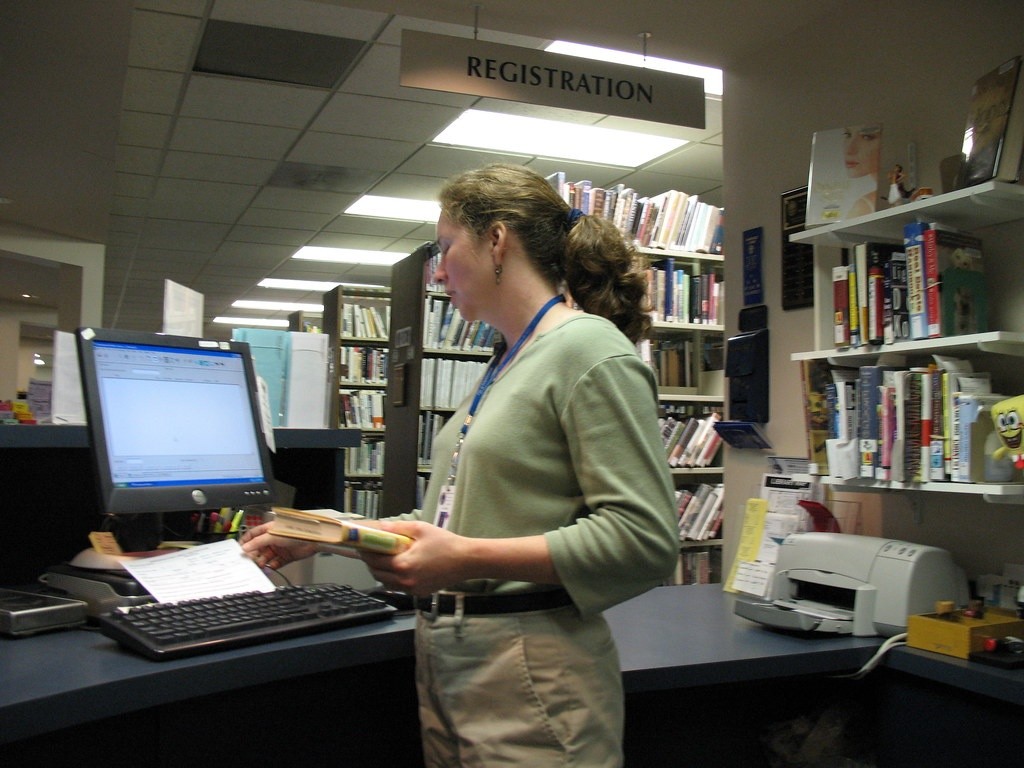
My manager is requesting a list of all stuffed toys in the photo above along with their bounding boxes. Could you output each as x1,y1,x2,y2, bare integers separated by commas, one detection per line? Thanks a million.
991,395,1024,470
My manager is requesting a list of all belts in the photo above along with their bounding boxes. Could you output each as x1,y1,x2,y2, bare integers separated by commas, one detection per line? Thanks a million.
416,589,575,614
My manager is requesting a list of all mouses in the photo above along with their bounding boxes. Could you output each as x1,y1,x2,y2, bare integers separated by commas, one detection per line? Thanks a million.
370,591,415,614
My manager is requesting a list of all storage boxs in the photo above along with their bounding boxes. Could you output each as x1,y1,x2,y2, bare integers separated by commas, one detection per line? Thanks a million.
907,609,1024,660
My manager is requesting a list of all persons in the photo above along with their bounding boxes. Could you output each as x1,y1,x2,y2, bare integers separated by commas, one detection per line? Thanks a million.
239,164,679,768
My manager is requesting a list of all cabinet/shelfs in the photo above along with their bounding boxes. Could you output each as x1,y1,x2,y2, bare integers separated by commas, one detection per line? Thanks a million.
790,180,1024,509
288,237,725,587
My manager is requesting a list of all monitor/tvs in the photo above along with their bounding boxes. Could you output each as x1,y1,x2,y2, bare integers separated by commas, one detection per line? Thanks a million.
71,327,280,579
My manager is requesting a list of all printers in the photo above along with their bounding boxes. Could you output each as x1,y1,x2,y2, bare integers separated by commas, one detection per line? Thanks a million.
733,532,971,637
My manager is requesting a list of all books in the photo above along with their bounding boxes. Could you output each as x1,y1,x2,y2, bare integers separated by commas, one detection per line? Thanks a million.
419,172,724,584
267,506,414,555
306,303,391,520
801,57,1024,482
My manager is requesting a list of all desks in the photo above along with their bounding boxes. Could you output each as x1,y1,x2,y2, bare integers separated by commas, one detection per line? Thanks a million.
0,426,1024,768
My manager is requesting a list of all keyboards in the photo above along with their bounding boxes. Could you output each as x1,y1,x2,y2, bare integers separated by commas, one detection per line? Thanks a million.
100,582,398,662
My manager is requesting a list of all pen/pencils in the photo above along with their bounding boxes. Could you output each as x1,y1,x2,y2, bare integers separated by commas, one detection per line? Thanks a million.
186,504,244,550
